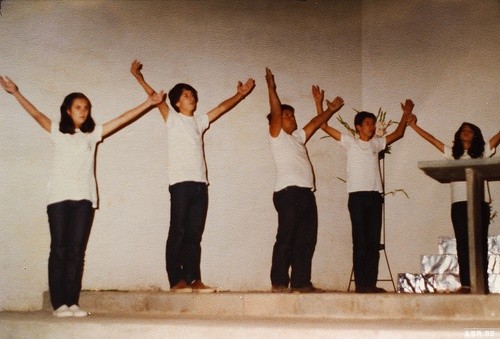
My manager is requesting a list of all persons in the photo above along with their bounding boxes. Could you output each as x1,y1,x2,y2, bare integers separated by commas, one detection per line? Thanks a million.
130,59,256,293
0,75,164,317
406,112,500,294
312,85,416,293
265,67,345,293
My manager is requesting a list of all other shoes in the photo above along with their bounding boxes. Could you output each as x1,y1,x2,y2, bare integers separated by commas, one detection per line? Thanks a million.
292,286,326,293
356,287,386,292
190,280,215,292
170,281,192,293
272,285,291,293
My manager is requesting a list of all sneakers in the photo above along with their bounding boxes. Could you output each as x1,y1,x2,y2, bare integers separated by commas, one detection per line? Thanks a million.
53,304,73,316
69,304,87,316
